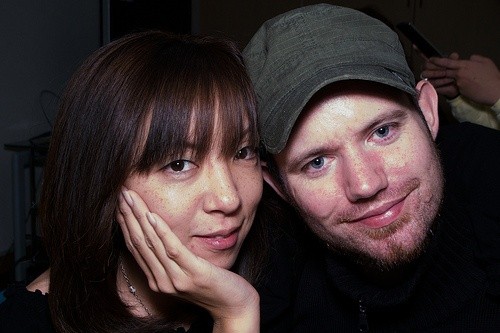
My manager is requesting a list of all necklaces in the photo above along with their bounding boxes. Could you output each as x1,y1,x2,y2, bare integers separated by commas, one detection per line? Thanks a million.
118,262,155,319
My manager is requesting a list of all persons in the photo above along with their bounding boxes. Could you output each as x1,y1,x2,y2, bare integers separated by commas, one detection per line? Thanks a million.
411,43,500,131
240,3,500,333
0,30,305,333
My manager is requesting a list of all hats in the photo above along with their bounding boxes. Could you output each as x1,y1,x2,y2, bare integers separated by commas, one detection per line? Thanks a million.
242,5,420,152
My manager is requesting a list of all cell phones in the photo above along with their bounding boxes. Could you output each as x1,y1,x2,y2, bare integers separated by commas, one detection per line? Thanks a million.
397,22,444,59
